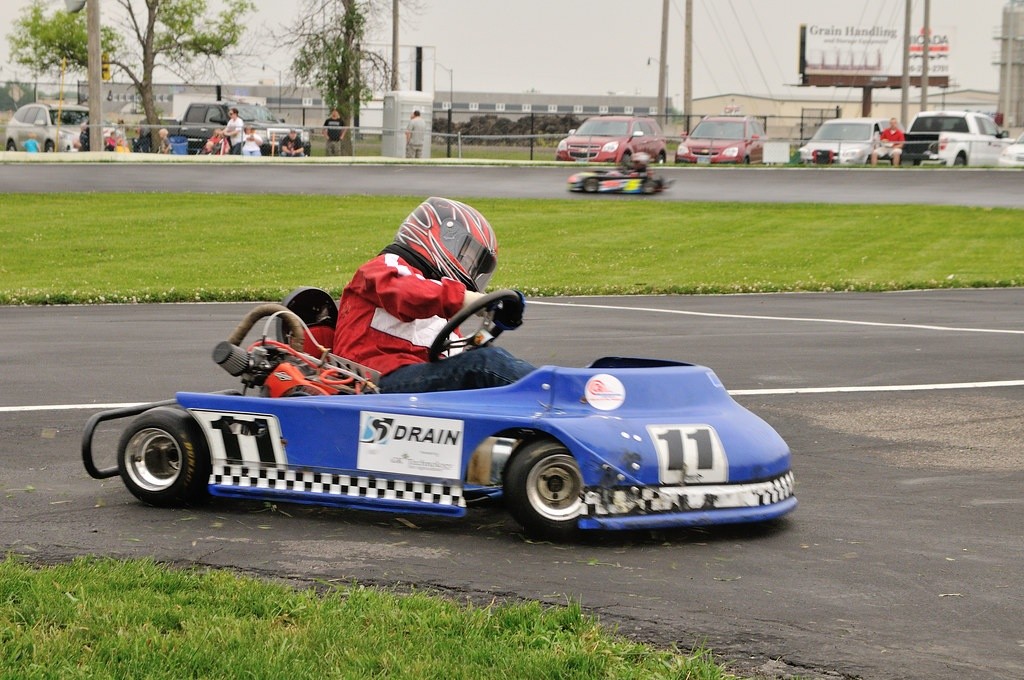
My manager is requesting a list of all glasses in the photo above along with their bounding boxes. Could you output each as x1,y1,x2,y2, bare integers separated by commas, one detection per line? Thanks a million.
230,112,236,116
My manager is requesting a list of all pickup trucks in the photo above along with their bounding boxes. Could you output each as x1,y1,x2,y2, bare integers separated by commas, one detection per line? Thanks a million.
138,102,311,156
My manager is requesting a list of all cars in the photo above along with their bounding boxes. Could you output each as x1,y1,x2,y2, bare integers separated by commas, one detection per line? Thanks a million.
906,111,1015,168
6,103,115,152
999,132,1024,167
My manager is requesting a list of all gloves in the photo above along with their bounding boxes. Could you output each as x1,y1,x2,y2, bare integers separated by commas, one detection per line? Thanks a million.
484,290,524,330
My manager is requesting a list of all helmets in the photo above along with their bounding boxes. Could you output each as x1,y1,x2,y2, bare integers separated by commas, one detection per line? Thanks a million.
397,195,499,294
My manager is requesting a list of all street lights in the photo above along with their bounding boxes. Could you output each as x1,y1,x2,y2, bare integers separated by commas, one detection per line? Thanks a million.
262,64,295,114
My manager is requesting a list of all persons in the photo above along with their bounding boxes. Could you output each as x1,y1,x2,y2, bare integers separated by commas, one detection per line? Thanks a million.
22,133,41,152
405,110,425,158
620,152,650,176
324,108,346,156
871,117,905,167
158,128,171,154
75,116,152,153
281,128,305,157
332,196,539,395
204,108,263,156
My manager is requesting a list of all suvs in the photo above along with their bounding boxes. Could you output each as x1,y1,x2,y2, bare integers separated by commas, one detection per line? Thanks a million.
675,115,766,165
555,113,667,165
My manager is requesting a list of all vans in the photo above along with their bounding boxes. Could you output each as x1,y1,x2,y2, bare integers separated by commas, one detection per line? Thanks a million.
798,118,905,166
